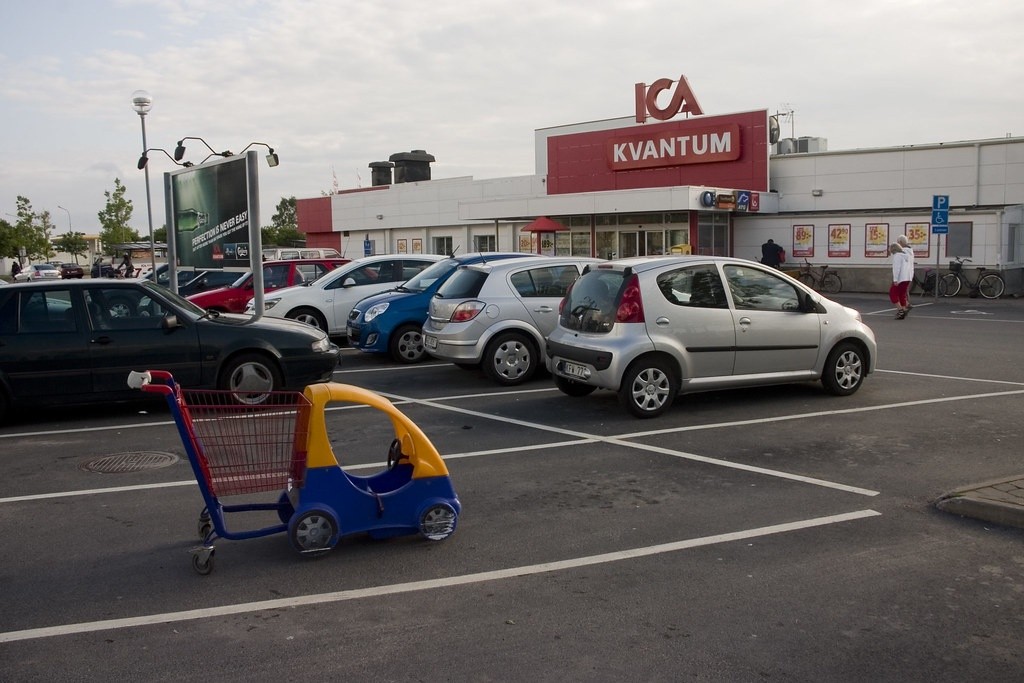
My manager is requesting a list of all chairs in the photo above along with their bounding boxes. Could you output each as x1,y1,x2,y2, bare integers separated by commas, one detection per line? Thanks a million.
553,267,577,295
689,272,717,304
88,301,111,329
538,268,562,295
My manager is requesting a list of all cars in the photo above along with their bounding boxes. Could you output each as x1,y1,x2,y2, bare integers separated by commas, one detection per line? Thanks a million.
183,257,379,315
57,263,84,279
245,253,456,336
0,277,340,421
421,256,693,387
147,263,304,294
546,254,877,420
13,264,63,282
346,252,547,363
0,279,119,317
91,264,111,278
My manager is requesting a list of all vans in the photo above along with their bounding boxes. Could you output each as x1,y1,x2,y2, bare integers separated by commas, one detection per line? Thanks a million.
262,248,344,262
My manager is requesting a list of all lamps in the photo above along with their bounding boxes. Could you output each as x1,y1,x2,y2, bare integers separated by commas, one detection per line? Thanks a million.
240,142,280,167
174,137,223,161
137,149,192,170
812,189,823,196
377,214,383,220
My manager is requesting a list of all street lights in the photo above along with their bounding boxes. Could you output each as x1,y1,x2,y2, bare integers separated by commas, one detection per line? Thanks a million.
58,206,71,235
130,89,158,285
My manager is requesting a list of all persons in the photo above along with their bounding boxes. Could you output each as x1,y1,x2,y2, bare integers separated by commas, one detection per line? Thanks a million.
12,261,21,283
117,255,134,278
761,239,783,271
889,242,906,320
897,235,914,312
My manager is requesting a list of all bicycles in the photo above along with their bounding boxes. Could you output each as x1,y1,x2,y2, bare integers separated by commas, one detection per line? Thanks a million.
939,255,1005,299
796,258,842,294
909,263,948,297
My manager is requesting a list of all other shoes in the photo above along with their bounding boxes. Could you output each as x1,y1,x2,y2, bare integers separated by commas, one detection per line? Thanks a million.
904,303,912,315
895,310,905,320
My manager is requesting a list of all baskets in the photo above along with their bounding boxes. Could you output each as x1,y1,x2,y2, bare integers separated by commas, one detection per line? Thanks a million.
800,263,809,274
949,260,961,271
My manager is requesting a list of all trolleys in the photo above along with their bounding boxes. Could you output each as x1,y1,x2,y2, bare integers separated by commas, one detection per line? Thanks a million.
106,269,126,279
126,370,460,575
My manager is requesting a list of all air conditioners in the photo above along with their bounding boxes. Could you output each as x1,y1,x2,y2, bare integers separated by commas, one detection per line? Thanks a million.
796,137,827,153
